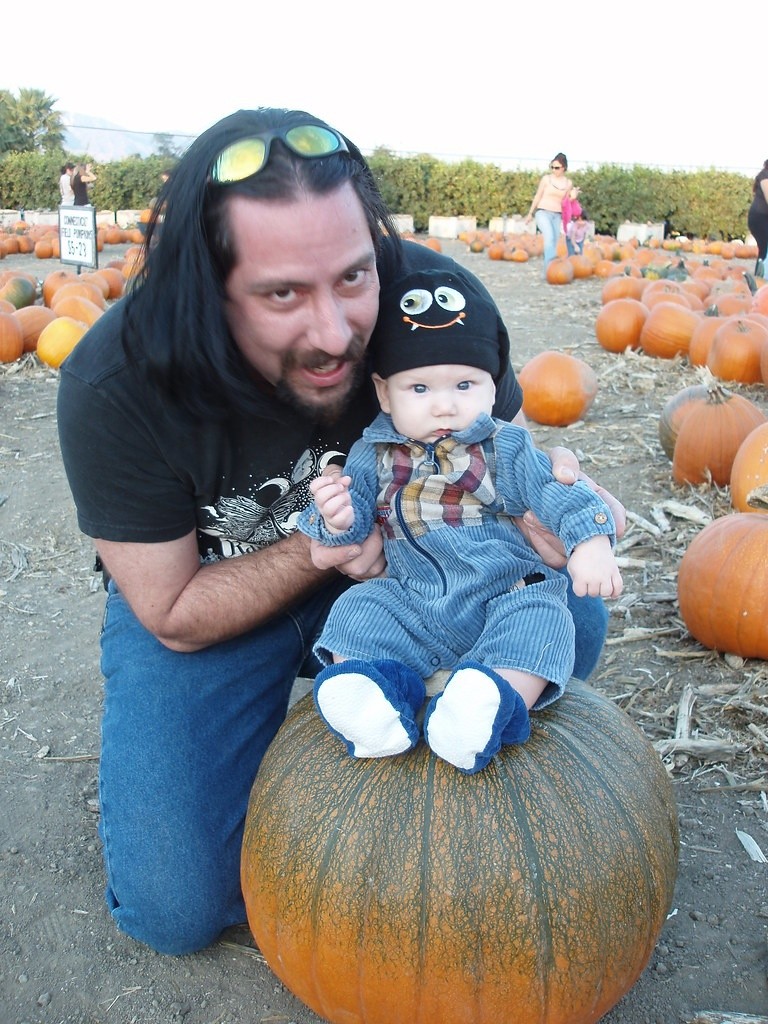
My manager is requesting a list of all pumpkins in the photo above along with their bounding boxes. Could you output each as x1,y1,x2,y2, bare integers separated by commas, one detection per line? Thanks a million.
0,209,164,367
241,675,680,1024
378,226,768,664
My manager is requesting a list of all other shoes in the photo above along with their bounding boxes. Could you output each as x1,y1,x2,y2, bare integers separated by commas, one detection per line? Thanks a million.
423,660,531,775
313,658,426,759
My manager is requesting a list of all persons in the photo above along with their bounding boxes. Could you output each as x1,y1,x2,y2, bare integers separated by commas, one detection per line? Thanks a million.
566,210,589,257
57,106,627,955
296,271,626,774
747,159,768,276
59,163,94,207
70,162,97,207
647,220,654,239
525,152,578,277
160,170,172,182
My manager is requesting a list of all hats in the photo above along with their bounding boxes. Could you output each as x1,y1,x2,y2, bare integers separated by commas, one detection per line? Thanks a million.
366,270,510,384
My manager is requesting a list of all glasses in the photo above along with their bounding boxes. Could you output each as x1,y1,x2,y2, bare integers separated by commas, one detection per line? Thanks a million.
551,166,563,170
202,122,351,187
